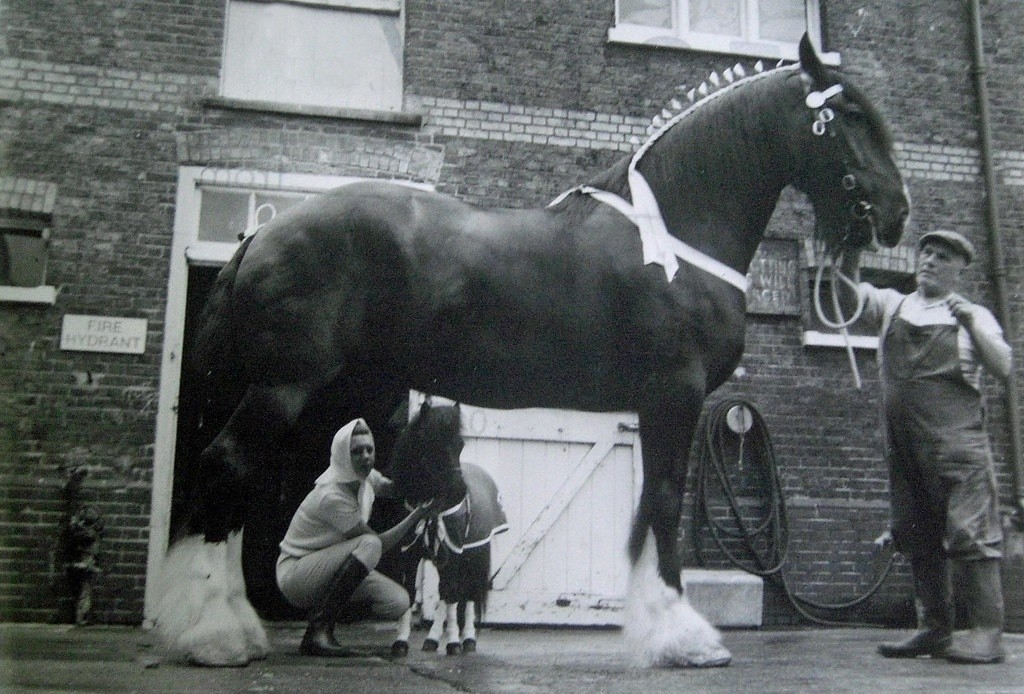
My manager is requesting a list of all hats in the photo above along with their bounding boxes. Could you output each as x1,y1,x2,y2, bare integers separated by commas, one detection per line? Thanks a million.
917,230,979,268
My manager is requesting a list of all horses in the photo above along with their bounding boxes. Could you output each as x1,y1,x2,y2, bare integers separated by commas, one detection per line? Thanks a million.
382,400,511,661
142,33,910,671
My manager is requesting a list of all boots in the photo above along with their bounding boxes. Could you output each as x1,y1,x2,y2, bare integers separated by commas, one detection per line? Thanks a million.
299,553,369,657
878,555,955,658
941,556,1003,661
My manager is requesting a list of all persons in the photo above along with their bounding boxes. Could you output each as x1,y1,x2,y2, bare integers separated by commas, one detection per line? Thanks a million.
275,419,436,656
838,231,1014,663
